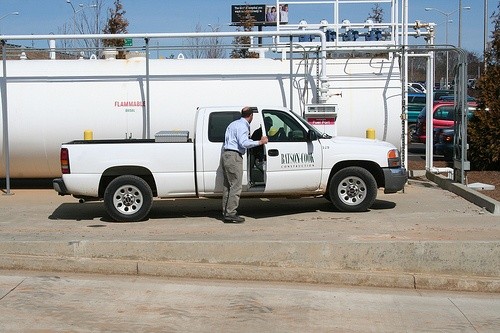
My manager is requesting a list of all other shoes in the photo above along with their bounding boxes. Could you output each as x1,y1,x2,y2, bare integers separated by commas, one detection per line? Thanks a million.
222,214,245,223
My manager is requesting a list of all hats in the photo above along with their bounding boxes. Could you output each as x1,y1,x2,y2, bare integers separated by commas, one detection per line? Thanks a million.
264,117,273,125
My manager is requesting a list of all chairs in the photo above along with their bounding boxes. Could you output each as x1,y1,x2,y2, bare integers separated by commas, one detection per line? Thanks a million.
251,148,263,182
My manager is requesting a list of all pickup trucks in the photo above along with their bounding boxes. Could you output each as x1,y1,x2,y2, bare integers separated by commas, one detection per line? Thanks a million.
53,104,408,222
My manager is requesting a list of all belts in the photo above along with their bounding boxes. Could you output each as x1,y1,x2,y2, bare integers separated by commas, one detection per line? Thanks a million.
224,149,242,154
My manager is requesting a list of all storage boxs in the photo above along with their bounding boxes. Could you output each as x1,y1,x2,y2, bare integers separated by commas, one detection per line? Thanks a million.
155,131,189,143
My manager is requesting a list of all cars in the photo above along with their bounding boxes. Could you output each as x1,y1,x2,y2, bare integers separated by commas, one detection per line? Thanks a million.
408,78,489,157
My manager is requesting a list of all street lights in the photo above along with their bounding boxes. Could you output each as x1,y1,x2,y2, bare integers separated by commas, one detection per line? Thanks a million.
208,24,224,57
425,6,471,89
433,20,453,91
67,0,97,49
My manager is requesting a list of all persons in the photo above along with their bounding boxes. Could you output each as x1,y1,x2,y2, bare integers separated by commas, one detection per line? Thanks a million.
280,4,288,22
267,7,277,22
222,107,269,223
252,116,283,159
